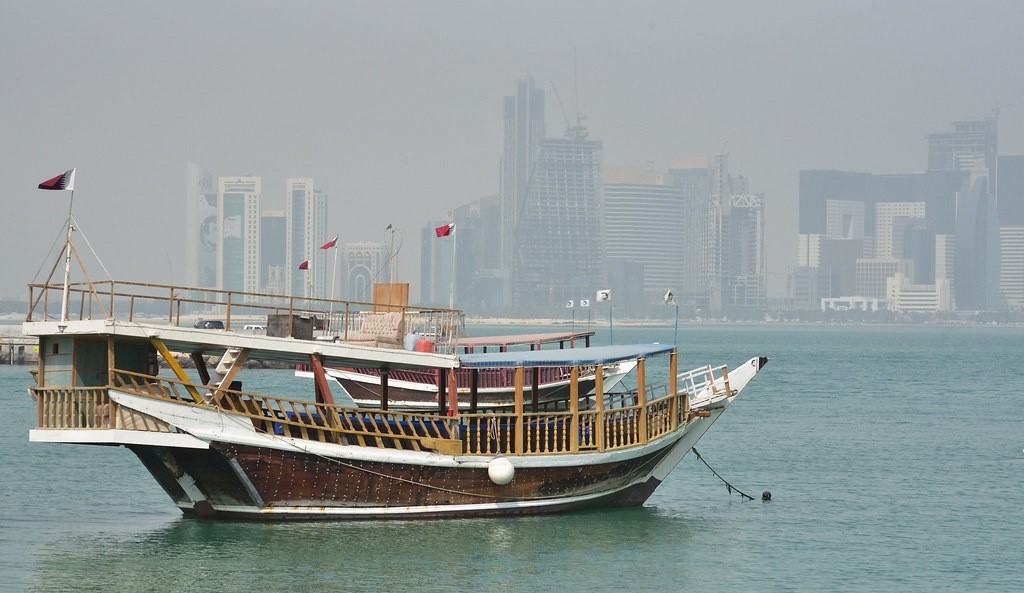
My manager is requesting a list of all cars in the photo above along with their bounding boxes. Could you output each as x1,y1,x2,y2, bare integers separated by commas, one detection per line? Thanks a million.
243,324,267,330
193,320,225,329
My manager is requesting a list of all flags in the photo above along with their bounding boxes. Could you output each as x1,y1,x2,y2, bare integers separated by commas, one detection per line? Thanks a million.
38,167,77,191
581,300,590,306
435,223,460,238
319,237,338,249
299,260,309,270
596,289,612,302
665,290,675,305
566,300,574,307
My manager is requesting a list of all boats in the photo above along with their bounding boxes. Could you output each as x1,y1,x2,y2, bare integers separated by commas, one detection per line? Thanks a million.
20,167,768,522
295,222,663,407
